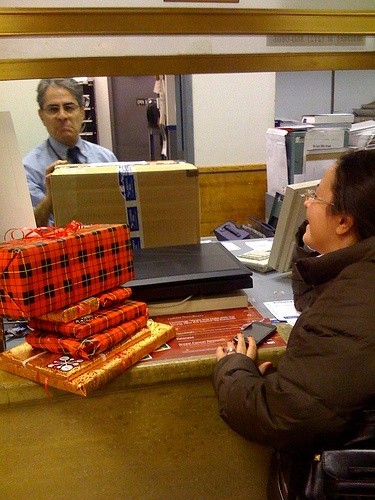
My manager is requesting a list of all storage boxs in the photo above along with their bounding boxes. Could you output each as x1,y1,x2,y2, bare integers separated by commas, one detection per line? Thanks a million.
50,160,200,248
302,113,354,129
0,221,135,318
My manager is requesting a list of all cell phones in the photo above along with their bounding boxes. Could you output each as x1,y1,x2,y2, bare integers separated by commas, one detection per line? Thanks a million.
233,321,277,347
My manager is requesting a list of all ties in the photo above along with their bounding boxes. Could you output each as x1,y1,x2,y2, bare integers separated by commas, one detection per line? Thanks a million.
67,147,82,166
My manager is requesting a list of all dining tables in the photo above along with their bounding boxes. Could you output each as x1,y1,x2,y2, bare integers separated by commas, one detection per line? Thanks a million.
274,448,375,500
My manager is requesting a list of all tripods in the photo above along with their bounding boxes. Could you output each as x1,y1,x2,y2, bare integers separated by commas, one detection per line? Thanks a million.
120,241,256,286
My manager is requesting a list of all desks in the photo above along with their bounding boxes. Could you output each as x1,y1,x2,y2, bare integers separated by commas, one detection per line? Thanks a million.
0,237,301,500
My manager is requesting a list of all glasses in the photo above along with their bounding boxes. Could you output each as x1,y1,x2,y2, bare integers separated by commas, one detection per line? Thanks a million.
43,104,80,115
304,188,335,206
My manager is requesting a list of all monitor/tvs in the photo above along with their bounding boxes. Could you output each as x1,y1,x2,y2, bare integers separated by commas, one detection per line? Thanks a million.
268,179,320,271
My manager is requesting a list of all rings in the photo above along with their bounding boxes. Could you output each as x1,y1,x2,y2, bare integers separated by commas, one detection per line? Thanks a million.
227,348,236,353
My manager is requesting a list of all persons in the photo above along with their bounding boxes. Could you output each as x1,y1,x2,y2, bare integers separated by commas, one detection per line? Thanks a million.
146,105,166,160
23,78,119,228
209,150,375,500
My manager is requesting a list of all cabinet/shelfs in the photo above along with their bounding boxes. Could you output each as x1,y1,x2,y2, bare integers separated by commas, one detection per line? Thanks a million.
79,81,97,145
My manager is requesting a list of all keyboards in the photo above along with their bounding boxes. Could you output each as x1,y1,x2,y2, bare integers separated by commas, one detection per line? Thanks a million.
234,246,273,274
